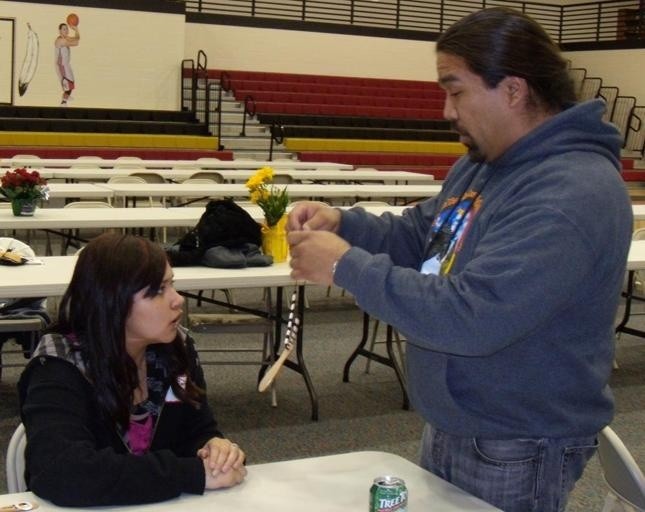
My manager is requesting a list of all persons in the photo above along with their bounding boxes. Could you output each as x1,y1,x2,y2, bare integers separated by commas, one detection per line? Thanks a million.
54,22,80,106
15,233,250,507
285,7,634,512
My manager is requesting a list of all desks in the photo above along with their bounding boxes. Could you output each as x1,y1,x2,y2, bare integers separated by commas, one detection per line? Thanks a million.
1,452,502,512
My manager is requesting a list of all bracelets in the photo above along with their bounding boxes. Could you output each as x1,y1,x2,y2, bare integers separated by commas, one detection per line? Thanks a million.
333,259,342,275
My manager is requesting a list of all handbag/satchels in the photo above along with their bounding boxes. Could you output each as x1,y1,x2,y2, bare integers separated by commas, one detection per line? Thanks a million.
167,197,273,266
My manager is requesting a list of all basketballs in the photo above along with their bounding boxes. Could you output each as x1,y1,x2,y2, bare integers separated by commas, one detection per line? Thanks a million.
65,14,79,27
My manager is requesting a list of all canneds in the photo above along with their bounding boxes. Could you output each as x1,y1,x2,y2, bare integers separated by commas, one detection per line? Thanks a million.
369,477,409,512
